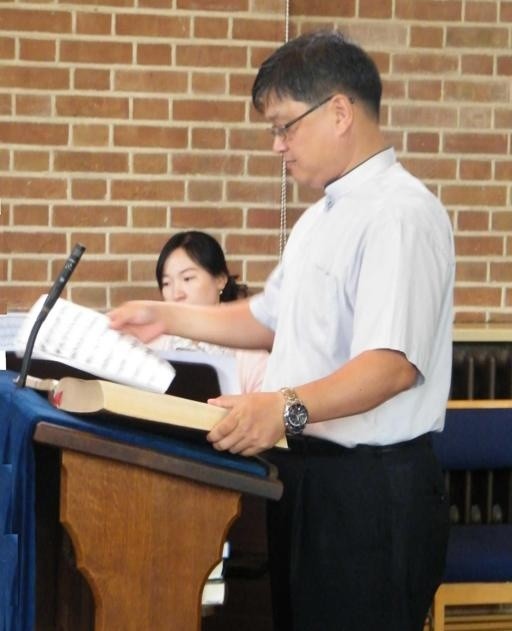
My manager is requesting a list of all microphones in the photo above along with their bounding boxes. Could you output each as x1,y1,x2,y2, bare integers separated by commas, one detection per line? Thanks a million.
15,243,85,391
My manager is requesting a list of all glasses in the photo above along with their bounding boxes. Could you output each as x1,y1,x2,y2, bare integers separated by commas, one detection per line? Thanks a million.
271,96,333,140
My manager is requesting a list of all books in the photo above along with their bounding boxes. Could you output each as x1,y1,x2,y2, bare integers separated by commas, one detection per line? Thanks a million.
12,373,289,449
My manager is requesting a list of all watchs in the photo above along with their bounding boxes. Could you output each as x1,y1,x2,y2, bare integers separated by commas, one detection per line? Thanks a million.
279,387,309,436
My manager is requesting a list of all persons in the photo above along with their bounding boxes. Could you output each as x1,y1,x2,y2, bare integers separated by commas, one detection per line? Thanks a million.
146,232,272,395
105,33,457,631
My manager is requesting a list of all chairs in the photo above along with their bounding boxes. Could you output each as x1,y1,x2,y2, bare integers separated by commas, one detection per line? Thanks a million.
427,404,512,631
